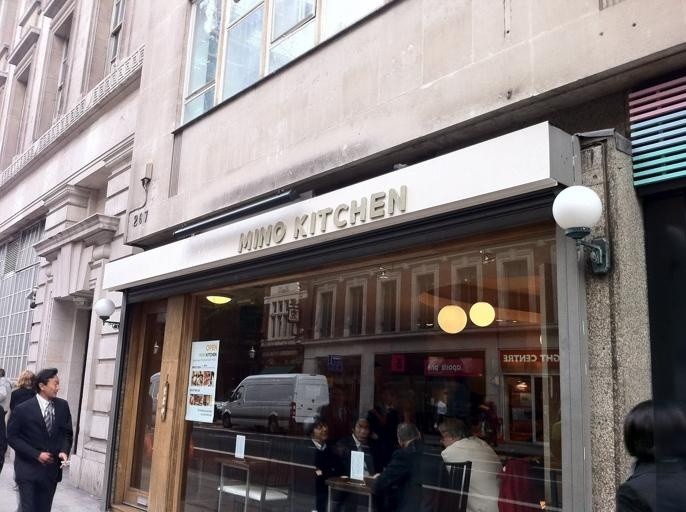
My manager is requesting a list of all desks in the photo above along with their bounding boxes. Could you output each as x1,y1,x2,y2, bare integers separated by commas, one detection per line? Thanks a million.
325,474,380,512
199,450,285,508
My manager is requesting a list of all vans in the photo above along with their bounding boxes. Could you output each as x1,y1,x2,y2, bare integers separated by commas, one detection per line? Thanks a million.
218,374,329,433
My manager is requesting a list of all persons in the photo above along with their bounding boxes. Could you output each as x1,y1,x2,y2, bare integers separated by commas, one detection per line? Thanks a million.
613,399,686,511
5,366,75,512
294,401,505,512
8,369,38,492
0,404,9,475
0,369,16,416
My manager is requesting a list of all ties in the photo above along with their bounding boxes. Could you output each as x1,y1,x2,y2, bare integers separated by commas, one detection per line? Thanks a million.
341,408,346,429
43,402,55,437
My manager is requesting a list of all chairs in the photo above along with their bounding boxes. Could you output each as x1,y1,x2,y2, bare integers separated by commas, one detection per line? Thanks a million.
431,457,543,511
214,437,300,510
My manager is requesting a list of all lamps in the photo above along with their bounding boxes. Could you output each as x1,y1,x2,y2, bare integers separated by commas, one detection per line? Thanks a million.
551,183,611,273
95,298,120,329
